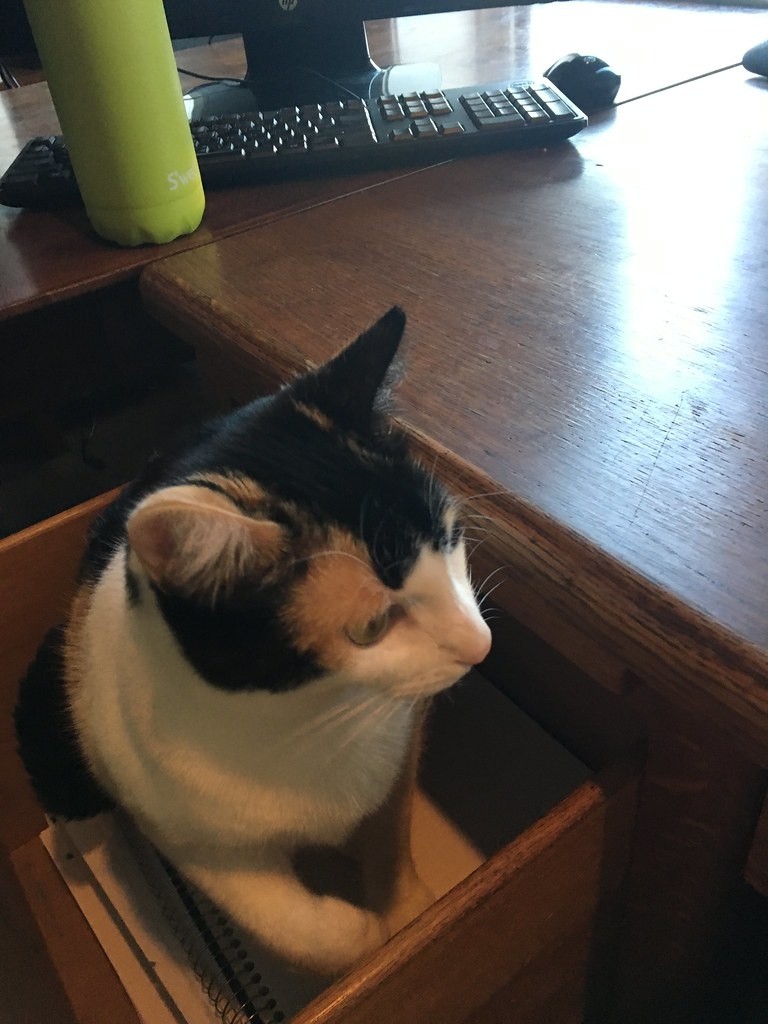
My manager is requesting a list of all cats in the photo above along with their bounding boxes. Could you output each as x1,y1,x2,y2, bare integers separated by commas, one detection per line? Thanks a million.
10,302,495,981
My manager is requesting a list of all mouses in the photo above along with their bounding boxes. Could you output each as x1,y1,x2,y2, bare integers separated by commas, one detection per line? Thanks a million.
543,51,620,110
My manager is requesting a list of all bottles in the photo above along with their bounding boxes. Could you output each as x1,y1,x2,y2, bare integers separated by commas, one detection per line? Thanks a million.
18,0,208,249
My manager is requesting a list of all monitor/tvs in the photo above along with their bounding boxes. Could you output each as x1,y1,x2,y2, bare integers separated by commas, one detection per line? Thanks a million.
0,0,572,90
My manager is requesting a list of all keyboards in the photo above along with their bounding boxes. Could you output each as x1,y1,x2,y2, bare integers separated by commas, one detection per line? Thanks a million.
2,75,590,205
